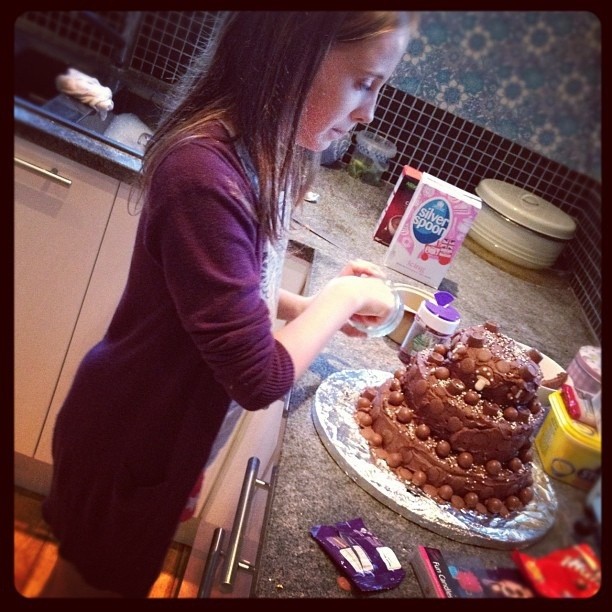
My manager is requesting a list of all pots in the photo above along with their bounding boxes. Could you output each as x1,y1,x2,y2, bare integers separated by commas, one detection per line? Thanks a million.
469,175,579,274
387,283,440,345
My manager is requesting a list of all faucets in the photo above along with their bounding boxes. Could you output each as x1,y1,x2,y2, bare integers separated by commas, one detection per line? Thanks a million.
72,11,144,71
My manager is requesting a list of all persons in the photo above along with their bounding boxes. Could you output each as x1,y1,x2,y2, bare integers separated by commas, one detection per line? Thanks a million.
43,11,415,597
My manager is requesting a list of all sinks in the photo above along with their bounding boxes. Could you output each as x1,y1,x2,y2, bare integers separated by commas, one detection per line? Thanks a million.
77,83,173,152
13,44,89,107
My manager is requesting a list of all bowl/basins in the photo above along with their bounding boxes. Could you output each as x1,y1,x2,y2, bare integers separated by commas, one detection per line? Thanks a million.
509,341,578,409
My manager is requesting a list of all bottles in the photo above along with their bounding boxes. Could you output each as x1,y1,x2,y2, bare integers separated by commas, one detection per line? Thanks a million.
348,129,397,184
400,290,461,365
349,274,404,338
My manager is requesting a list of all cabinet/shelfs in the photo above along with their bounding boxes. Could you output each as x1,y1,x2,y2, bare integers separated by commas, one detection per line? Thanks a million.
15,131,120,462
163,399,285,599
35,181,313,522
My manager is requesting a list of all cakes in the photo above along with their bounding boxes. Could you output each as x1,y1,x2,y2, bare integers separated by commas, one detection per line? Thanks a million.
355,320,546,517
